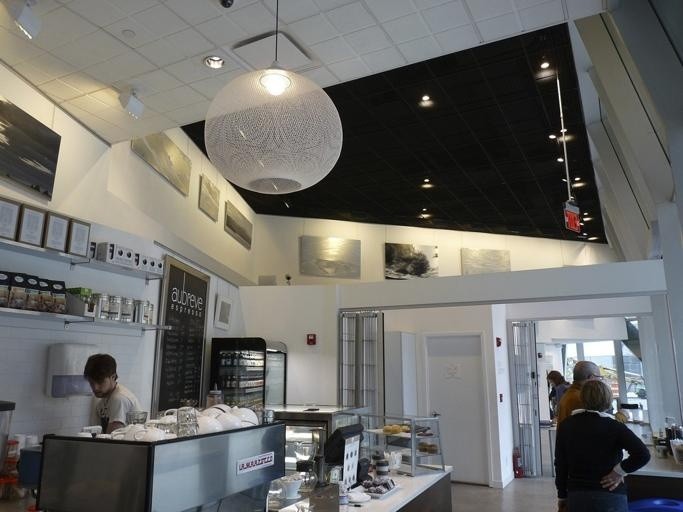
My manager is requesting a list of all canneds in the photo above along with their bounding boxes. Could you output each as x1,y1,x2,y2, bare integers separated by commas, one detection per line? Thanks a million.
91,293,154,325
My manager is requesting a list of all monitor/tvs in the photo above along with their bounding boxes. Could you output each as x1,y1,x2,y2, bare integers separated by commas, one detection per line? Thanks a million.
315,423,364,462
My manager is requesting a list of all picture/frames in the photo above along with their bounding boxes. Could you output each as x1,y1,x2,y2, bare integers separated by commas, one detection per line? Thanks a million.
213,294,232,330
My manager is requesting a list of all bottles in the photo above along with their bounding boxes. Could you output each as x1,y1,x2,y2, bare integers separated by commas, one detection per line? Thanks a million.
295,460,319,492
336,480,349,512
0,440,20,504
205,383,225,410
91,293,155,326
655,427,668,459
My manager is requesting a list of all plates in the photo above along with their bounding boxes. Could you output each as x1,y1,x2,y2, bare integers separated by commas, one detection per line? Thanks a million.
363,483,402,500
349,491,371,504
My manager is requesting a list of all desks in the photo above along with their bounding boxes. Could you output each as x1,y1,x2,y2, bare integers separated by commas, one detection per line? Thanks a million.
539,426,559,477
615,442,682,501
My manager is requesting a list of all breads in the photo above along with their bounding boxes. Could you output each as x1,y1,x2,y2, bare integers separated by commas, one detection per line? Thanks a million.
383,424,438,455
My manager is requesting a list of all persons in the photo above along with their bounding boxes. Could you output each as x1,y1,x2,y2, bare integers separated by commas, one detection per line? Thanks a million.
552,378,651,512
83,353,143,434
554,361,604,424
546,370,573,428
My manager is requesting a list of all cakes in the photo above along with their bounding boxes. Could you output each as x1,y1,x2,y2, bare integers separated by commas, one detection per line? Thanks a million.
363,478,395,495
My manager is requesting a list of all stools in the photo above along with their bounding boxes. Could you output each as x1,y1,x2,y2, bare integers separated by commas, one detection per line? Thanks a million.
628,497,683,512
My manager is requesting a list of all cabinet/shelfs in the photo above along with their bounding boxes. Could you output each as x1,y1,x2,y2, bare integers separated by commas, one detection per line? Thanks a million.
260,405,359,478
1,234,174,334
358,410,447,480
210,337,288,416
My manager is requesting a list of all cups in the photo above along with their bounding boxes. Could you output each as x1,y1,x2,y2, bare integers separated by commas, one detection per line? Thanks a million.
668,440,683,466
375,460,390,479
14,433,25,455
26,435,39,449
74,400,260,442
371,453,385,476
280,476,303,498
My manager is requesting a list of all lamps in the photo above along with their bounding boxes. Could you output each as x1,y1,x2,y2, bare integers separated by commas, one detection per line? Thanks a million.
203,1,347,194
